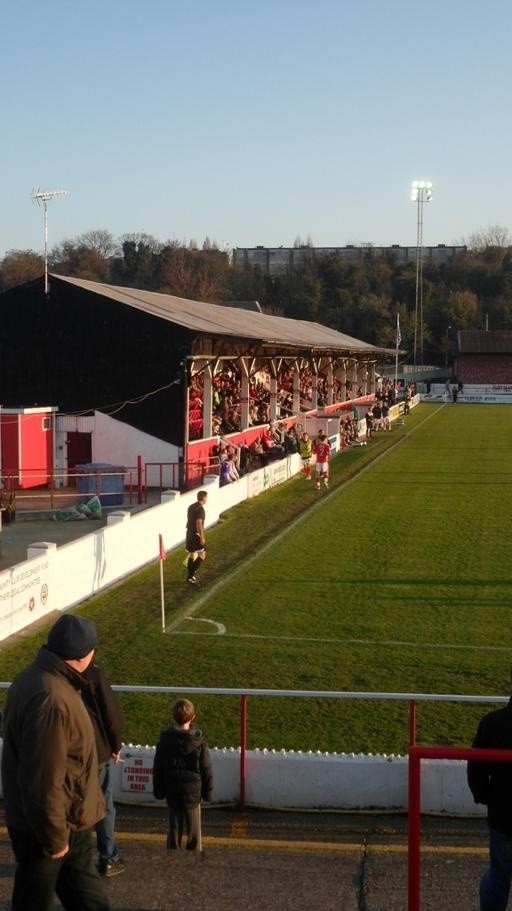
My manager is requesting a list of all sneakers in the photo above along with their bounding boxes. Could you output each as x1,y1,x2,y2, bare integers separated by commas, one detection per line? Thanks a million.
304,475,329,490
98,859,127,877
185,576,197,583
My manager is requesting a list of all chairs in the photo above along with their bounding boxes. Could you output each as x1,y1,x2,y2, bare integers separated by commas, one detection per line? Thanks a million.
189,368,368,441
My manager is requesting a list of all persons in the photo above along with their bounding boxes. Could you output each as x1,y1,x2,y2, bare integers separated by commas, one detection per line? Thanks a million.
466,692,511,911
72,647,129,878
152,698,214,852
1,613,112,910
185,491,209,585
188,357,465,493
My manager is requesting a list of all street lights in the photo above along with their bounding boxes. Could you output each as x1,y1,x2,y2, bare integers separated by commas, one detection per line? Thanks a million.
410,179,433,396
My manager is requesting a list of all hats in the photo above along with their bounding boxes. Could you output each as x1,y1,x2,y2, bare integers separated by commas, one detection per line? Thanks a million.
47,614,97,660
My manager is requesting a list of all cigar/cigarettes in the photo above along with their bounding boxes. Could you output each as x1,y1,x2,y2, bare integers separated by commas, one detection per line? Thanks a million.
114,758,127,764
